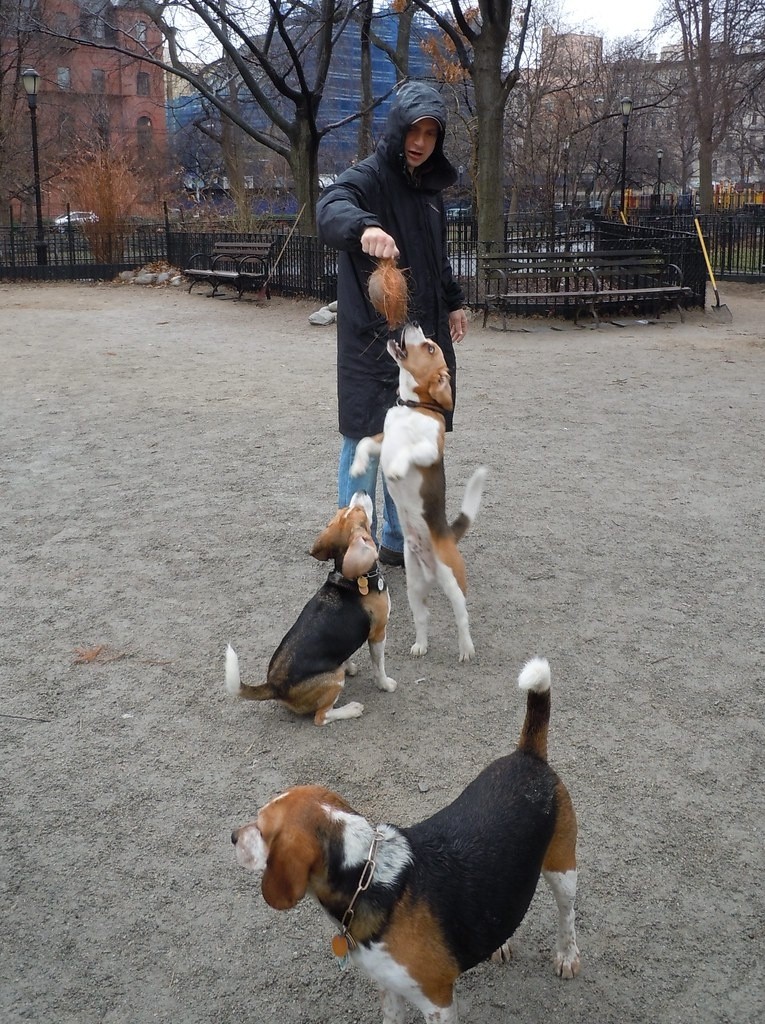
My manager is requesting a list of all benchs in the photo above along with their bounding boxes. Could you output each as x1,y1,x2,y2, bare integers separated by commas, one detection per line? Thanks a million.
476,250,691,333
183,241,268,300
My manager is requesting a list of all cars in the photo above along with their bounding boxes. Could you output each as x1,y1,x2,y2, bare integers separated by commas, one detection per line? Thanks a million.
446,209,468,222
262,179,293,197
554,202,563,210
51,211,99,234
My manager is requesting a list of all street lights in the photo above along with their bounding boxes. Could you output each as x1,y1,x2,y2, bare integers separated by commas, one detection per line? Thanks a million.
195,125,216,201
620,96,633,215
458,166,464,222
564,137,569,210
656,149,664,204
20,68,49,267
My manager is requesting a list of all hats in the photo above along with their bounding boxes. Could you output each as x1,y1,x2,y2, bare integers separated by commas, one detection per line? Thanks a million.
410,115,443,132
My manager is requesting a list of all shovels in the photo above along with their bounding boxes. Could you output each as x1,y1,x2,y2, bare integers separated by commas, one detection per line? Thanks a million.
254,203,307,306
695,218,733,324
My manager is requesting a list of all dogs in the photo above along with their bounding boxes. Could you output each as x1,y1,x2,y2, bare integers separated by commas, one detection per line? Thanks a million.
229,654,581,1024
223,320,487,726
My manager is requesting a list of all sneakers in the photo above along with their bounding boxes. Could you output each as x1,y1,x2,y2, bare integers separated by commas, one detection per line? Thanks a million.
378,545,404,565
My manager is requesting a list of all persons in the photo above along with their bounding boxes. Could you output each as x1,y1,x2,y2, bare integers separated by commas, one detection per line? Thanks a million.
316,82,468,567
